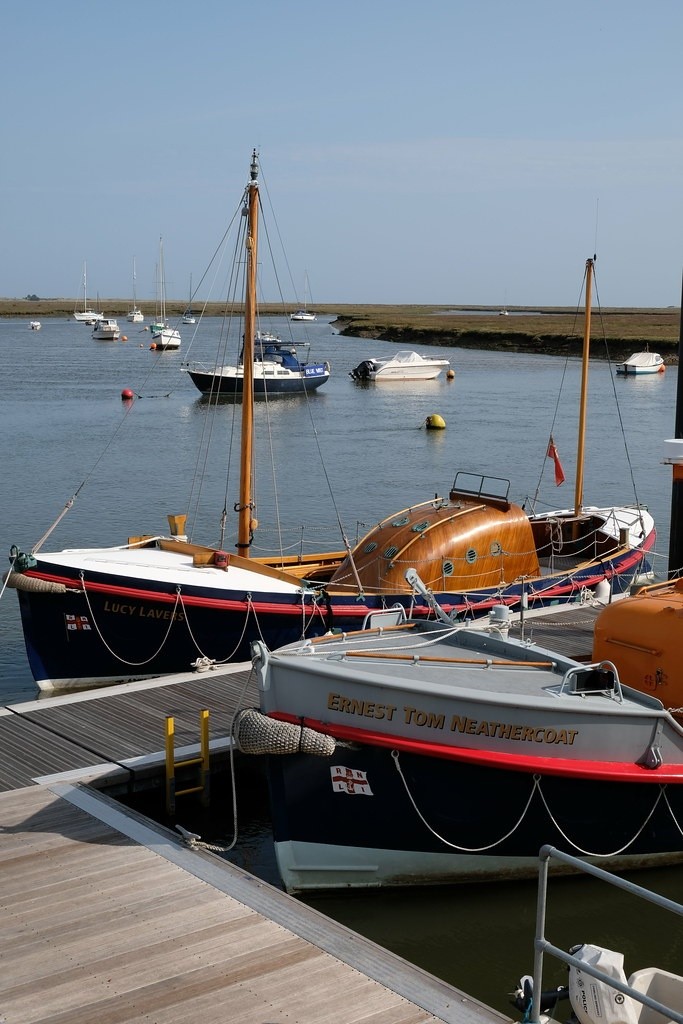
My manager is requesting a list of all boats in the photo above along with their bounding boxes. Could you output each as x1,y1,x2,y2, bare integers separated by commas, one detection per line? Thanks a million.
499,310,508,315
348,350,457,384
234,355,683,898
1,147,661,698
27,321,41,331
179,330,330,393
92,319,122,340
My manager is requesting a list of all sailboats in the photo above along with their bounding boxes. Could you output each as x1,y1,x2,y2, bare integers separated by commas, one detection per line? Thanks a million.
124,255,146,322
74,259,106,321
150,236,182,349
179,273,198,324
291,276,317,321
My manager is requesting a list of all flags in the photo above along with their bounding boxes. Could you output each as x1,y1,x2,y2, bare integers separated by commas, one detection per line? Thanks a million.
547,438,565,486
